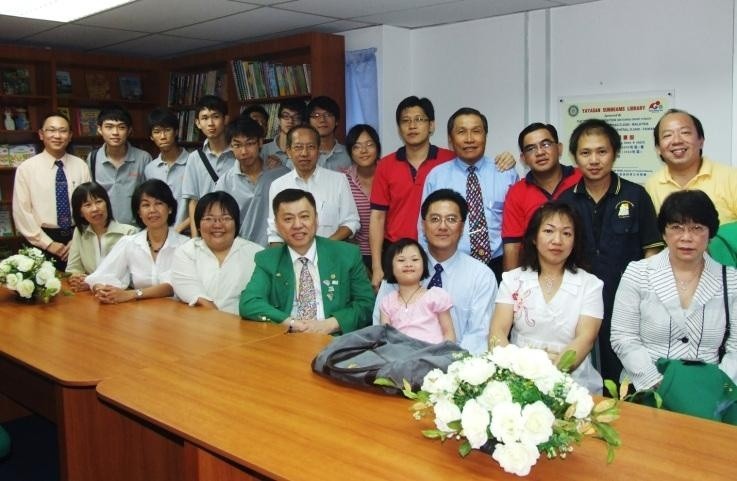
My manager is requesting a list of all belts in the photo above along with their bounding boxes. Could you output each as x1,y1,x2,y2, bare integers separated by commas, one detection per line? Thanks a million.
53,230,71,237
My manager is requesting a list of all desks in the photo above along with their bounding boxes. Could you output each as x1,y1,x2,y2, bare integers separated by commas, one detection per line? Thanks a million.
1,279,292,480
95,327,735,480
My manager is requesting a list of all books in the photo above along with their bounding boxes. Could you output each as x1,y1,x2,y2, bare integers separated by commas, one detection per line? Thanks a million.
167,55,315,147
1,65,155,240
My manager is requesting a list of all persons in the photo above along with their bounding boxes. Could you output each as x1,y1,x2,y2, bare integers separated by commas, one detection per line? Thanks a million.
11,96,735,425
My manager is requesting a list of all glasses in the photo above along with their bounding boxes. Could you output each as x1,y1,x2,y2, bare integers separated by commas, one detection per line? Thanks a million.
428,215,461,225
668,224,704,236
525,141,554,155
281,111,302,120
199,216,234,223
401,116,428,124
308,112,332,118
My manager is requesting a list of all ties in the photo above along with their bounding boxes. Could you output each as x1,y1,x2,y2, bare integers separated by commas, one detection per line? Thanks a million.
54,159,72,229
296,258,316,320
428,264,444,289
465,166,491,265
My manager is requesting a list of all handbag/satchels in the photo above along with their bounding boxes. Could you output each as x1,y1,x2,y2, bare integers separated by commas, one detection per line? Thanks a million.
311,324,471,396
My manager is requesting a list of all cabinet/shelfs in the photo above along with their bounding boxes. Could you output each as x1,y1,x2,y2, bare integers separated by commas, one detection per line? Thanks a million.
164,44,235,142
229,36,345,147
0,45,159,240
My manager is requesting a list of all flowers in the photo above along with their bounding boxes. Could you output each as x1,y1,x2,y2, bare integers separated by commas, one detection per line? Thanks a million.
373,335,666,481
0,247,76,309
